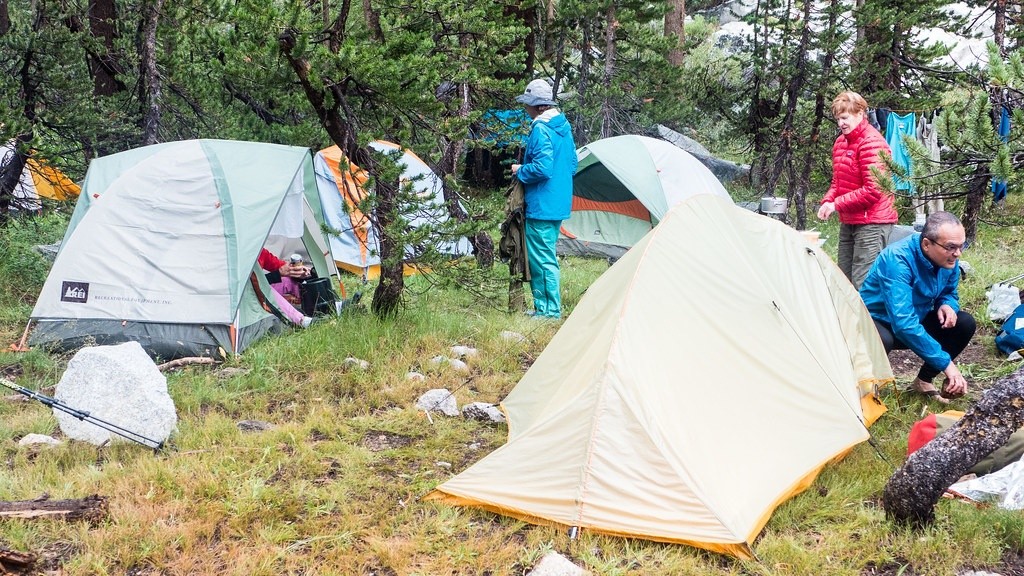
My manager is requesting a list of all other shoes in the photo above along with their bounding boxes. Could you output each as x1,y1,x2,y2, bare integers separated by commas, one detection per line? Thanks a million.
300,316,318,330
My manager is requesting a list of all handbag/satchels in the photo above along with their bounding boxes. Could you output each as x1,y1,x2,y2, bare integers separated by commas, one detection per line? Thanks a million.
995,303,1024,361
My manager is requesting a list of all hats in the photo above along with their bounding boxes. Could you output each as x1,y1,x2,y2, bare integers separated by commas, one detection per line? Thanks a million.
515,79,558,106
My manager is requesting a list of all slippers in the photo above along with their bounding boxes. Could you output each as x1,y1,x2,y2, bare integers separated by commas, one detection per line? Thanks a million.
906,377,950,404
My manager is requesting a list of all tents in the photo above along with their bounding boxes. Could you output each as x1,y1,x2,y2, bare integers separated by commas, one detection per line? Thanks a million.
0,137,82,216
462,106,540,187
645,124,753,188
314,138,476,284
555,134,735,263
420,205,900,563
19,138,344,361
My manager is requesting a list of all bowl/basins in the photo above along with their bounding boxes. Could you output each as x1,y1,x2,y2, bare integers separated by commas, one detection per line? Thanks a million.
802,232,819,244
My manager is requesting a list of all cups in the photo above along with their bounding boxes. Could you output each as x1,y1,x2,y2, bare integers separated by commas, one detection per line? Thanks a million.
291,254,303,272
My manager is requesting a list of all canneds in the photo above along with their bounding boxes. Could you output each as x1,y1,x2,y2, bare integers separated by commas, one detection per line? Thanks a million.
290,254,303,271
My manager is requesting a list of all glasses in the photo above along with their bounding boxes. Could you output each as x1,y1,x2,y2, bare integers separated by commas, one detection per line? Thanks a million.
929,238,970,253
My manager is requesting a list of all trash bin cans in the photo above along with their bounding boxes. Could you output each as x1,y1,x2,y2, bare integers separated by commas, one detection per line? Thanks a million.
302,277,336,315
762,197,788,223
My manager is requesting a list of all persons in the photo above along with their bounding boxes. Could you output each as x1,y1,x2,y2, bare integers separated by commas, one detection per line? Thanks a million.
258,246,314,329
510,80,580,318
858,210,979,407
816,90,899,291
